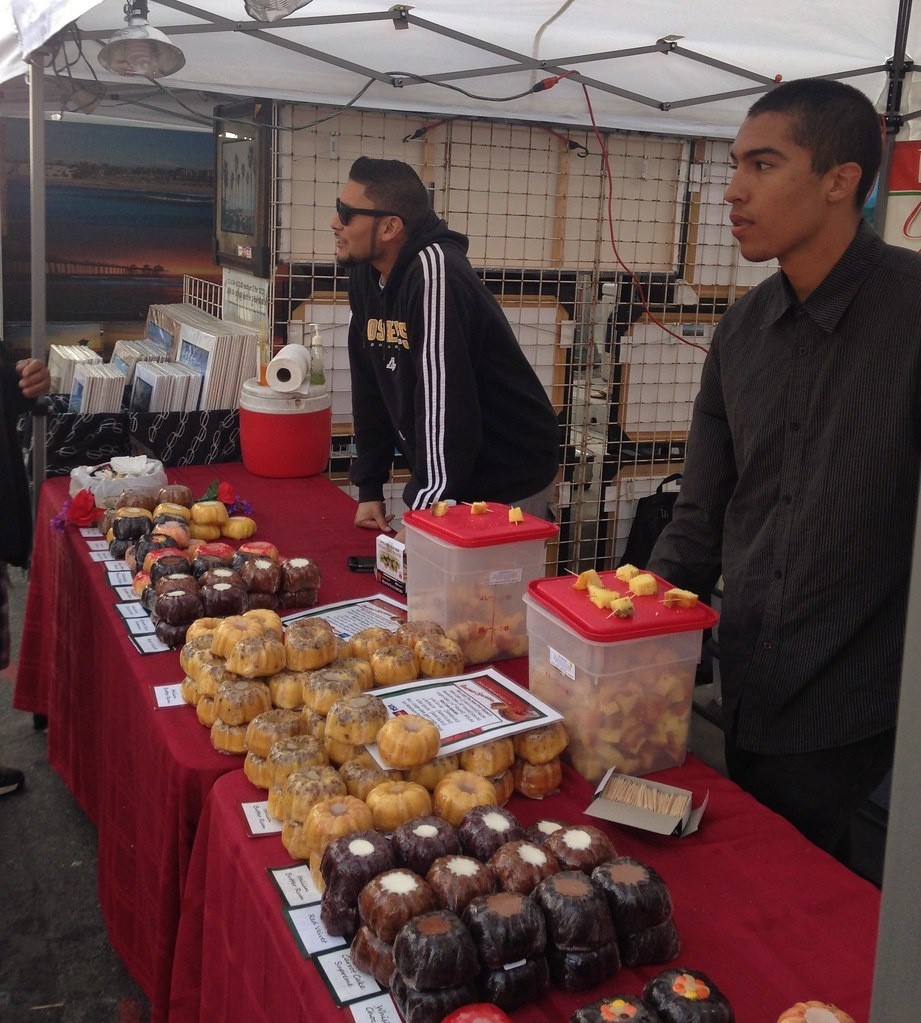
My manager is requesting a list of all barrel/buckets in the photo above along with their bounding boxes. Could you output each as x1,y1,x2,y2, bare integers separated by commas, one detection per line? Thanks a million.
239,377,333,477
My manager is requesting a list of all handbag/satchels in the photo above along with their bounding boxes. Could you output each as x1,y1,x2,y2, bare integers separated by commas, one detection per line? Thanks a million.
618,473,683,569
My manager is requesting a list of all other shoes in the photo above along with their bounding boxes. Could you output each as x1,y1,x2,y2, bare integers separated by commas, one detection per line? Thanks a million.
0,767,25,796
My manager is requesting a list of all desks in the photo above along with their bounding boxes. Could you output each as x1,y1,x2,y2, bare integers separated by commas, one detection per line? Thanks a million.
13,464,884,1023
17,388,241,479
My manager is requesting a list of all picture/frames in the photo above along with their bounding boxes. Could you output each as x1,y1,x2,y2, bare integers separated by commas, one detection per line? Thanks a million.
283,285,573,577
271,100,689,278
680,140,778,300
610,309,724,447
601,457,685,569
48,302,256,414
212,98,272,279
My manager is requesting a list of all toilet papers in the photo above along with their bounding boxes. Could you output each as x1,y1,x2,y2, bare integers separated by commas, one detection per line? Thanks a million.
265,342,312,395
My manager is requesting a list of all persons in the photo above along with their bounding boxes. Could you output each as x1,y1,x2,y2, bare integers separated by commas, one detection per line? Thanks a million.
330,155,562,570
647,78,921,890
0,338,50,796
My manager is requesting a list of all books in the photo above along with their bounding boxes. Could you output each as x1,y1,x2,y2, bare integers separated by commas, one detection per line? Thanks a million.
47,302,257,418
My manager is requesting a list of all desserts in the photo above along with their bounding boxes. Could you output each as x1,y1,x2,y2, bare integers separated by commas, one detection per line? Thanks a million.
99,485,321,642
182,608,569,884
319,809,732,1022
777,999,852,1023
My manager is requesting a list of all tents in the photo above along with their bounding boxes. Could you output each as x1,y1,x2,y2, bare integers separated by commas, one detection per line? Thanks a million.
1,0,921,1023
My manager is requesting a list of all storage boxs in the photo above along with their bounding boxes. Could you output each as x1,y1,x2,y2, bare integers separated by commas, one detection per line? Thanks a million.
522,572,719,785
402,501,560,666
373,535,408,595
240,377,331,478
582,765,709,839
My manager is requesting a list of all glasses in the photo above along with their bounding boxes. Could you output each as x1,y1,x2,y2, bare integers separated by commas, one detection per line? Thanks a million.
336,198,409,227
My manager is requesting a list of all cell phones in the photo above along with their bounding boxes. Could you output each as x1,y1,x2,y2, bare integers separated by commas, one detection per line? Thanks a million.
347,556,376,572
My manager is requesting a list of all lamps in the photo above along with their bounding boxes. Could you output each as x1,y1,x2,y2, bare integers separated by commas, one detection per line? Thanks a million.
97,1,187,80
